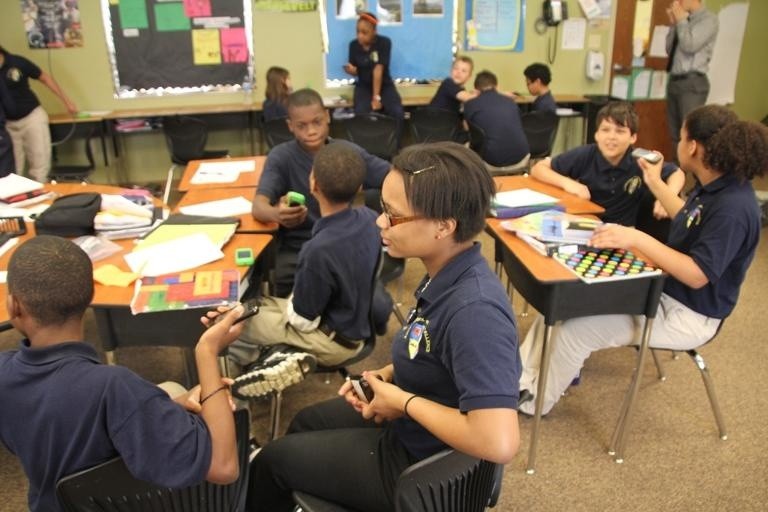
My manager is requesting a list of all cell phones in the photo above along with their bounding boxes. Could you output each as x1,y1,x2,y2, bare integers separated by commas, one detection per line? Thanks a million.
235,247,254,265
209,298,260,327
632,147,660,164
288,192,305,207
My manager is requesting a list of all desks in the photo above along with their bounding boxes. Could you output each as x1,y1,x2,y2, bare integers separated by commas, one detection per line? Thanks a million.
2,154,289,392
484,174,607,318
484,215,668,473
0,94,592,165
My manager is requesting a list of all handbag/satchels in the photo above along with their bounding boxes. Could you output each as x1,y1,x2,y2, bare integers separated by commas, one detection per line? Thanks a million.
34,192,101,237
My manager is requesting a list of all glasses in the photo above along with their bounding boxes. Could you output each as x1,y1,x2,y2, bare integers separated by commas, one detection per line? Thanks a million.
380,195,425,227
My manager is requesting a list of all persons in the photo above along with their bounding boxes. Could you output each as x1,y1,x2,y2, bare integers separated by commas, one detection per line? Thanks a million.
666,0,719,167
0,234,249,510
344,12,404,120
262,65,294,122
0,45,80,182
251,88,393,295
530,98,686,229
519,107,768,419
246,140,524,512
428,54,556,174
225,141,386,404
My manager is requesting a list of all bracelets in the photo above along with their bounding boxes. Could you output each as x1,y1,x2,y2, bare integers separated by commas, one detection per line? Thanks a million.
404,395,418,417
371,95,382,100
199,386,227,403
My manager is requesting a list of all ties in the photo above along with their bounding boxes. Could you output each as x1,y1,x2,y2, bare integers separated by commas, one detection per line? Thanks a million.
665,32,679,72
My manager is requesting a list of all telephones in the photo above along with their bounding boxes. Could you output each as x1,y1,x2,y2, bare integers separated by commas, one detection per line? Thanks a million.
543,0,568,26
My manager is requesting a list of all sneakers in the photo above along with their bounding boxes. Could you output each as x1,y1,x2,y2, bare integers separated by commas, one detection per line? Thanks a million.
232,343,318,400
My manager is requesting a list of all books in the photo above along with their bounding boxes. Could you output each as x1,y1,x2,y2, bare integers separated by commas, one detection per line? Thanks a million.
487,186,663,286
1,172,240,278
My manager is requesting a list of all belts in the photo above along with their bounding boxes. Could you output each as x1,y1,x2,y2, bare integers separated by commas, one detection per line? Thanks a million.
668,72,705,80
317,322,368,350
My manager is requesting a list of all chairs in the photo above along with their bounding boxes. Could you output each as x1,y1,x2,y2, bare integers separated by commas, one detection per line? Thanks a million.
270,245,406,441
294,446,503,512
56,409,250,512
607,316,728,456
